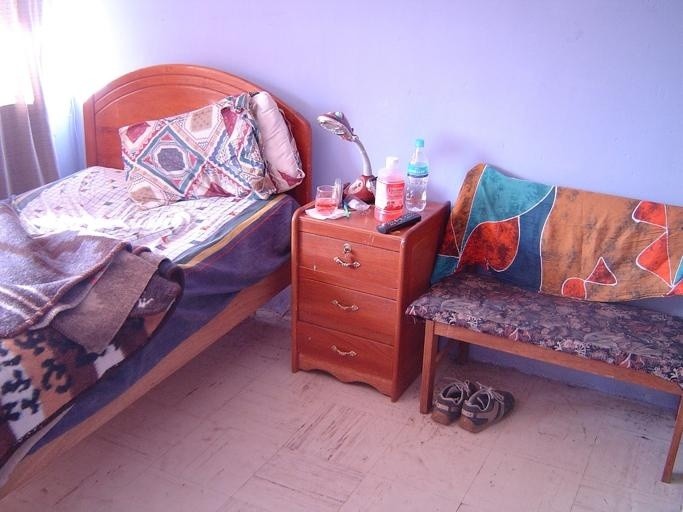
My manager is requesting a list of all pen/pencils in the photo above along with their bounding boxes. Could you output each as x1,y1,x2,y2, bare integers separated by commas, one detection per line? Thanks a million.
343,204,350,217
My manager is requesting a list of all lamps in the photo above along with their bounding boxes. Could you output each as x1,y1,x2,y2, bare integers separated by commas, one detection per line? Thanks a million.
316,112,378,200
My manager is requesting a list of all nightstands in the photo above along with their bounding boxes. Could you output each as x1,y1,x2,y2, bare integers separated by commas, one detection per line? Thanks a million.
289,181,451,403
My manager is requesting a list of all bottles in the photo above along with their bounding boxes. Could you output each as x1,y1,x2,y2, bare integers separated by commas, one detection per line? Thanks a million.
375,138,429,221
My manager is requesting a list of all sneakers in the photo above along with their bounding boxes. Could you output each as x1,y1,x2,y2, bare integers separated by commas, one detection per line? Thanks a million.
460,386,515,434
432,380,477,425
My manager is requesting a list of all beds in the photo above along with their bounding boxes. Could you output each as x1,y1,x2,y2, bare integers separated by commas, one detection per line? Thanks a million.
0,64,313,493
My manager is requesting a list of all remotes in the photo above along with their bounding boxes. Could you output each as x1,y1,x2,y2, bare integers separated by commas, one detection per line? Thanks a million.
376,213,423,234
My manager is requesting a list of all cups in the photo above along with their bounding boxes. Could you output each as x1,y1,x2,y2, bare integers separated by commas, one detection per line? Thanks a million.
315,185,338,217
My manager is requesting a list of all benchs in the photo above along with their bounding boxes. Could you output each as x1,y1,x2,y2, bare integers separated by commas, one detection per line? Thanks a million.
406,163,681,482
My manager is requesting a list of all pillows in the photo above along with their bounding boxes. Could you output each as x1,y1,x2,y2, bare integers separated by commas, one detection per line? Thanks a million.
117,88,305,206
